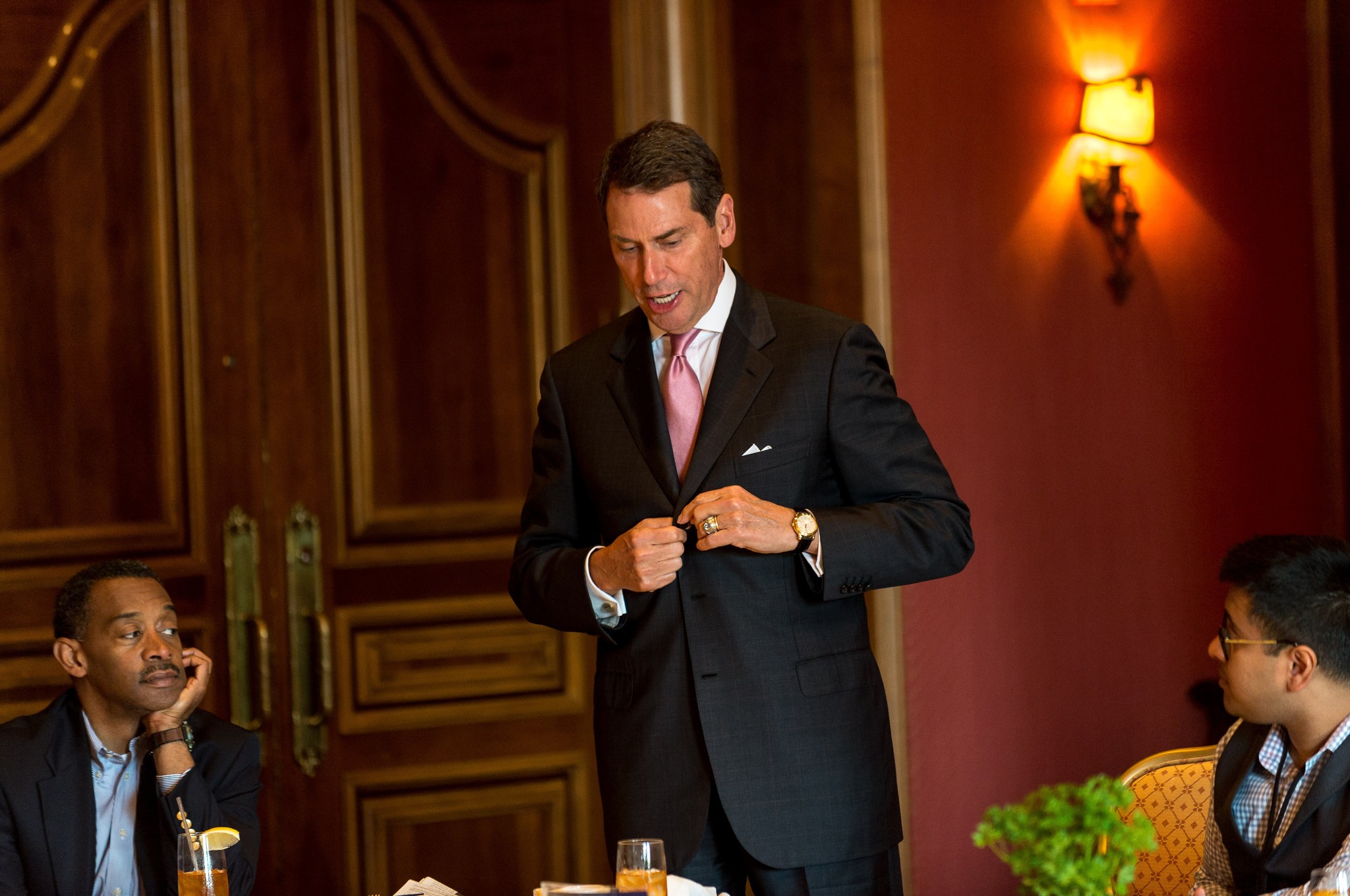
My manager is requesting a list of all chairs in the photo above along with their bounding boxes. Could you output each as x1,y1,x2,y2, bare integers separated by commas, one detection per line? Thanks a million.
1094,744,1220,896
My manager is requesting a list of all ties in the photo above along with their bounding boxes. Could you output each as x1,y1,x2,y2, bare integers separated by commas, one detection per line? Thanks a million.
660,329,705,489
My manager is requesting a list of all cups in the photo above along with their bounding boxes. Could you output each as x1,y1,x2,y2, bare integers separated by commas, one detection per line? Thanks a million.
616,839,667,896
177,831,230,896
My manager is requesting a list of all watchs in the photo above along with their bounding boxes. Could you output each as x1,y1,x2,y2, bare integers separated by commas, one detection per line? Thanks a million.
791,508,818,554
146,721,195,756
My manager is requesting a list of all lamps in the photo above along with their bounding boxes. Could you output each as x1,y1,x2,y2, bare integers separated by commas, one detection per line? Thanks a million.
1076,75,1156,296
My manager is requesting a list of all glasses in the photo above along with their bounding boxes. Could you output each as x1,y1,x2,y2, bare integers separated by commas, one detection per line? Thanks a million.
1216,626,1300,663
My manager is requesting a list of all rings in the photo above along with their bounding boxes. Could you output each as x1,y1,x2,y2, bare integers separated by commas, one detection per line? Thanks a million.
703,515,720,534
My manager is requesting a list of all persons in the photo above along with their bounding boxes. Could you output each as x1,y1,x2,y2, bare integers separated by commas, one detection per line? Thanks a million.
1190,529,1350,896
505,120,973,894
0,559,264,896
